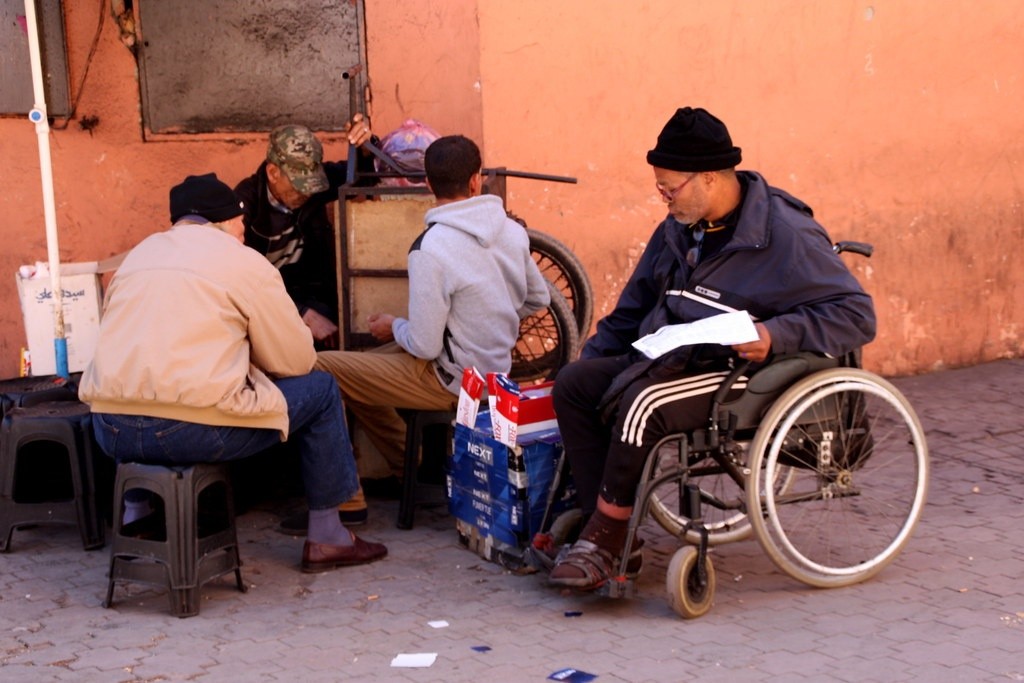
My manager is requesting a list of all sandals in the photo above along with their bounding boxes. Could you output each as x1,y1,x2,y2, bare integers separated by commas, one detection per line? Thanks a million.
550,539,645,587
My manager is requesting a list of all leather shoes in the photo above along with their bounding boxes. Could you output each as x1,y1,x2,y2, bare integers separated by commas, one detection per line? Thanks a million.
303,537,386,572
124,534,146,561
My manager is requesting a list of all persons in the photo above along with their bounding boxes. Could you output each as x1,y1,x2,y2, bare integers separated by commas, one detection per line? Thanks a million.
278,135,551,535
78,172,389,575
231,113,384,511
533,107,877,587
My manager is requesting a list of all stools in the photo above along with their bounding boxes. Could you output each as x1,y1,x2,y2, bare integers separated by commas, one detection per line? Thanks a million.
0,375,248,619
393,408,456,531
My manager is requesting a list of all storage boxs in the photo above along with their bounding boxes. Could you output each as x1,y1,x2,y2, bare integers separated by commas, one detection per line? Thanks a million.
14,251,128,376
446,378,579,574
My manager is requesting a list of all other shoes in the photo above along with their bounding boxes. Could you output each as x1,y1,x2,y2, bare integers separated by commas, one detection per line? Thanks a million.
281,508,368,533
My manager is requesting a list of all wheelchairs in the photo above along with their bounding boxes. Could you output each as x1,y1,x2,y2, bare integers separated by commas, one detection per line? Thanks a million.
516,240,931,620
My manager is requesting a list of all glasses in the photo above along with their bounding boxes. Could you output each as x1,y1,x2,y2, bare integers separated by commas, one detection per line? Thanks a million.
655,174,696,201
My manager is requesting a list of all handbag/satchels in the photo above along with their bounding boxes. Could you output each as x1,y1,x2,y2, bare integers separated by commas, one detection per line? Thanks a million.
375,120,440,188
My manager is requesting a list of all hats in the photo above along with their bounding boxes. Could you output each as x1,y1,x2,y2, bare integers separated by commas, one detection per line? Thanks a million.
170,172,251,226
647,106,742,172
267,124,331,193
